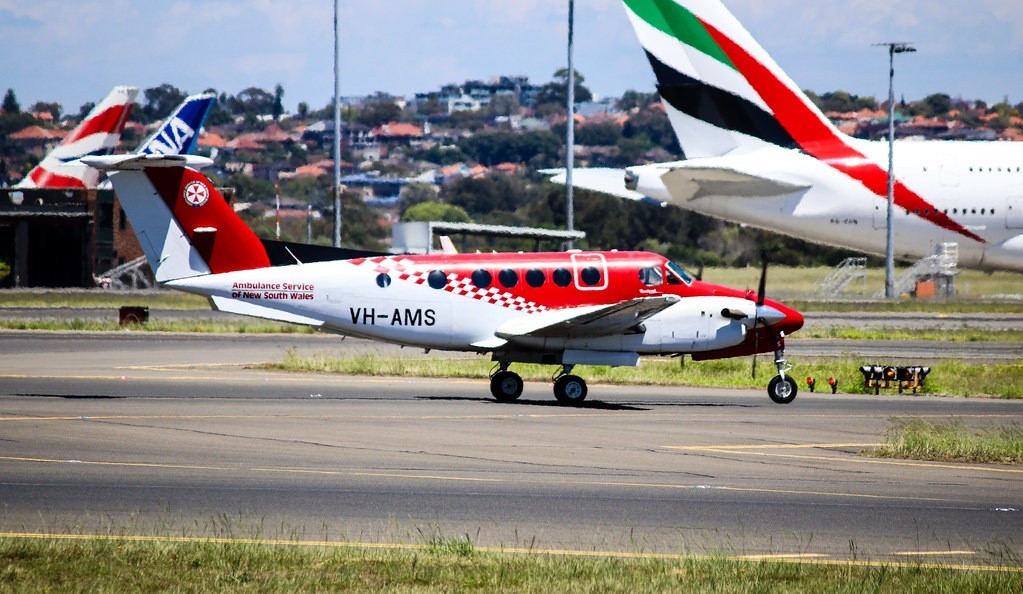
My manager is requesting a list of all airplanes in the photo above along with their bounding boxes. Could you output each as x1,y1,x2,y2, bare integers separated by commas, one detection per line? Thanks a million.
536,0,1023,278
106,168,804,406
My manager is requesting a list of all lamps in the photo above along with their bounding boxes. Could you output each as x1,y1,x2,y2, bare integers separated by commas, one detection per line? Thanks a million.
860,365,931,395
807,377,816,392
826,377,838,393
